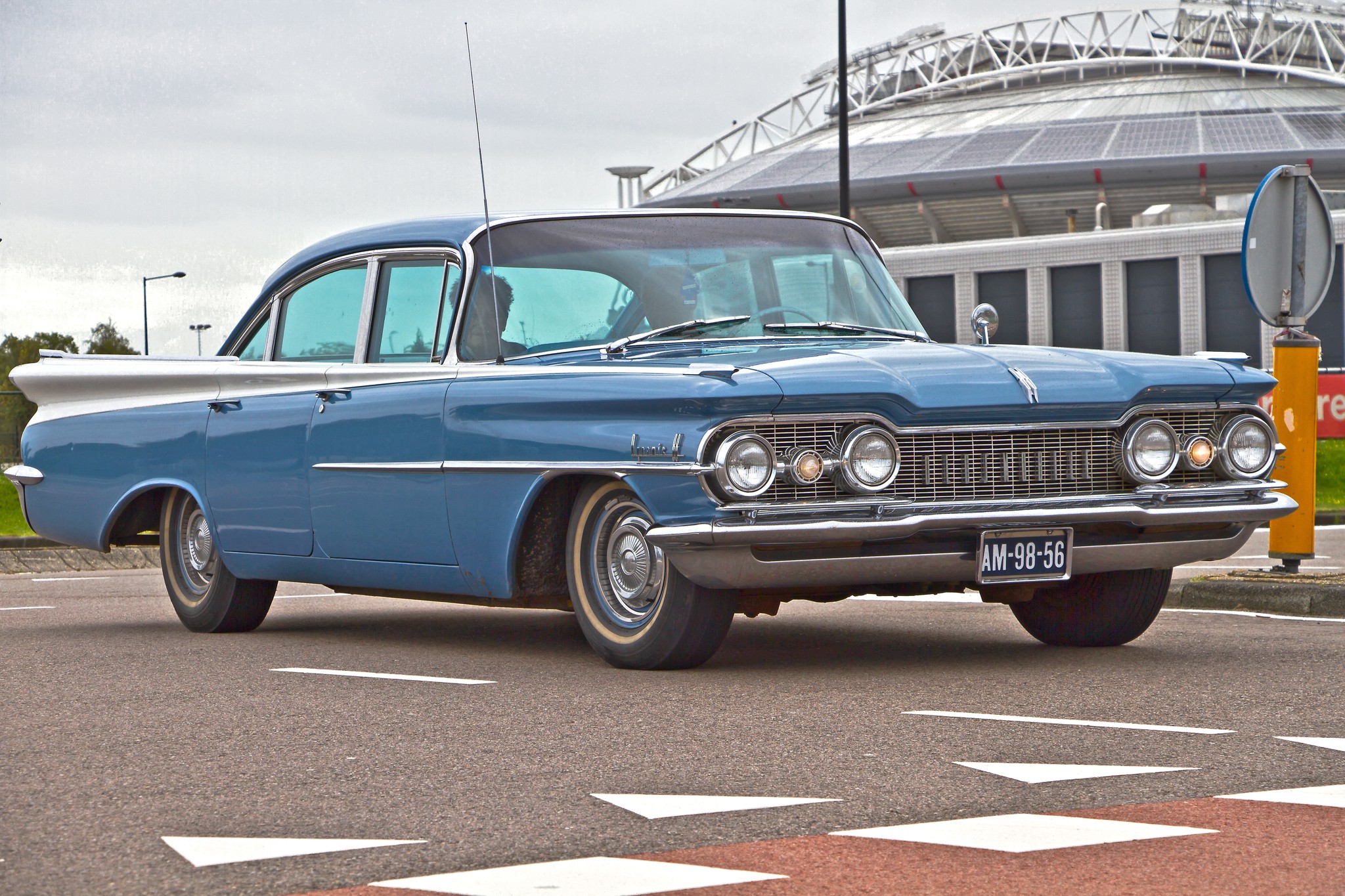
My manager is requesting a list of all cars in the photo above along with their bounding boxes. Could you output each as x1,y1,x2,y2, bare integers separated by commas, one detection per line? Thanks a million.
5,22,1300,671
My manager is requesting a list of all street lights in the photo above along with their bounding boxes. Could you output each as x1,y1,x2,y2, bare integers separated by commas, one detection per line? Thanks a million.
142,272,184,357
190,324,212,356
389,330,398,355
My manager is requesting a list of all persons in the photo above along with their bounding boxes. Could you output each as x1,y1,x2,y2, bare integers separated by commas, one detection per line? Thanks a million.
641,263,701,334
448,273,530,365
